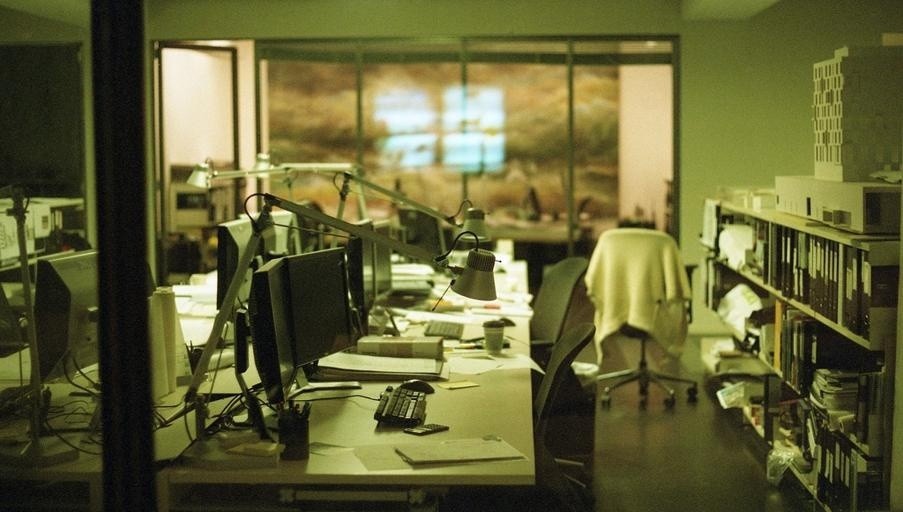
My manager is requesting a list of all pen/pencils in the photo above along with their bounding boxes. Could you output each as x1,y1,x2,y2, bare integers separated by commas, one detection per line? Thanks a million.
276,400,311,423
458,335,486,344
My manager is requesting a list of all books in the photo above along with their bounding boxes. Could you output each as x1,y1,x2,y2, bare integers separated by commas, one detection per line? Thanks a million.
358,335,444,362
719,212,900,512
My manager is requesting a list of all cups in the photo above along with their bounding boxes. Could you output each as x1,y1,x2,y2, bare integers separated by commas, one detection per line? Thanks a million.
277,420,308,460
482,320,504,355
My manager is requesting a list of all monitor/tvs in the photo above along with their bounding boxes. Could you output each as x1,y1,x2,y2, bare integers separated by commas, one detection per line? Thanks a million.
398,204,454,270
369,220,405,336
214,216,276,312
278,247,362,401
349,218,376,322
34,251,100,431
236,257,298,412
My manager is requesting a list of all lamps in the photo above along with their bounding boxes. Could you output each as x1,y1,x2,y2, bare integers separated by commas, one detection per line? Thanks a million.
186,158,304,255
252,153,369,219
329,171,490,243
183,191,501,436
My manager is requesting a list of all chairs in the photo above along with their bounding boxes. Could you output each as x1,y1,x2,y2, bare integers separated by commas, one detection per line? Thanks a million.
531,257,589,352
0,284,30,386
536,322,596,489
584,228,698,410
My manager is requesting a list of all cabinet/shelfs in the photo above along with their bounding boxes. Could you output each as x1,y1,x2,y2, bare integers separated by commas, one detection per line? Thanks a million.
717,202,900,512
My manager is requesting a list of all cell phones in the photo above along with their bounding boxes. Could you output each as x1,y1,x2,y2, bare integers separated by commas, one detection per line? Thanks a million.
403,423,450,436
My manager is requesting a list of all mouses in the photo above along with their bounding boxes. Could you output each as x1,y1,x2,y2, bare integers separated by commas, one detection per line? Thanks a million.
495,315,515,327
401,375,435,395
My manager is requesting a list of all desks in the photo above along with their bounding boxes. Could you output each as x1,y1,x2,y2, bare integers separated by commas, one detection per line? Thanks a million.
0,314,194,512
156,260,536,510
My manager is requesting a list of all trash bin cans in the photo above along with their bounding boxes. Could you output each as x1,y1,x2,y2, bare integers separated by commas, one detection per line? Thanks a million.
558,363,598,456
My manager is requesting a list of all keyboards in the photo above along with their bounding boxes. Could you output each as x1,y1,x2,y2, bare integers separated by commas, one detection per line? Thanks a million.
374,386,426,427
426,321,463,341
